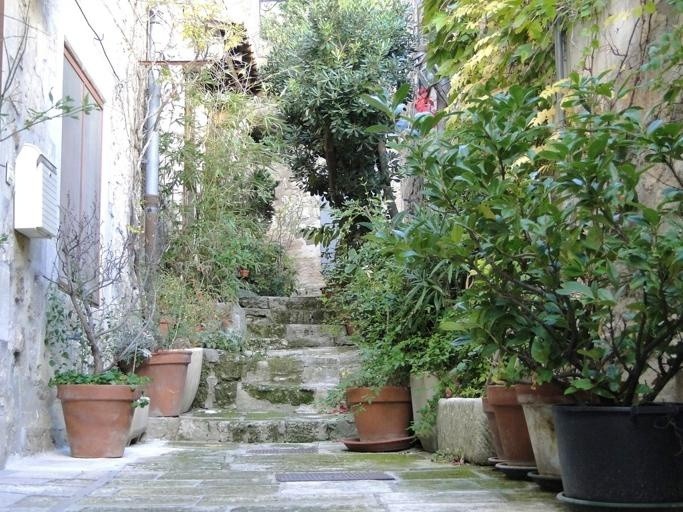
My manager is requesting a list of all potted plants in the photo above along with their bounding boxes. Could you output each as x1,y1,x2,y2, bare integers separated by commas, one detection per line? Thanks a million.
42,199,205,460
341,69,683,512
